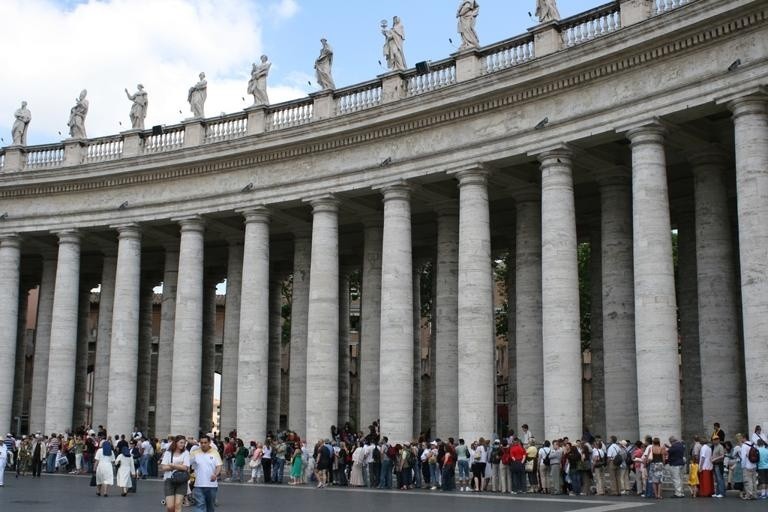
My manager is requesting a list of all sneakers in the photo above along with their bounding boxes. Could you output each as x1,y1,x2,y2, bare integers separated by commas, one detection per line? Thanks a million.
712,494,716,497
717,494,723,497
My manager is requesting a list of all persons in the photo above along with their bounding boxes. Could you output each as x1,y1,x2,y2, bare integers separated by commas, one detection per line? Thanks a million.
125,83,148,129
1,418,768,512
383,15,406,71
70,90,89,138
11,100,31,144
314,38,335,89
456,0,479,48
536,0,561,22
191,71,207,116
249,54,271,106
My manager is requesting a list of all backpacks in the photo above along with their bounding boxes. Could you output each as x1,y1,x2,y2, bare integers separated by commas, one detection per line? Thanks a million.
491,446,501,464
613,447,622,466
543,448,551,466
745,442,760,463
385,444,397,458
406,450,416,465
502,446,511,465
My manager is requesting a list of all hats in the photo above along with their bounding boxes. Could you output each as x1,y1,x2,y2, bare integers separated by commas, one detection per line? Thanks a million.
429,440,437,446
401,441,410,446
618,440,627,448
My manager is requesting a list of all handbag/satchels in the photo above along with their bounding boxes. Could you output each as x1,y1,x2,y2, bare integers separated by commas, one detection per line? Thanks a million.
525,459,533,471
249,459,260,468
473,451,481,459
171,470,190,483
466,446,470,458
646,446,653,464
595,460,604,467
373,444,380,461
625,452,633,466
429,454,436,464
90,476,96,486
